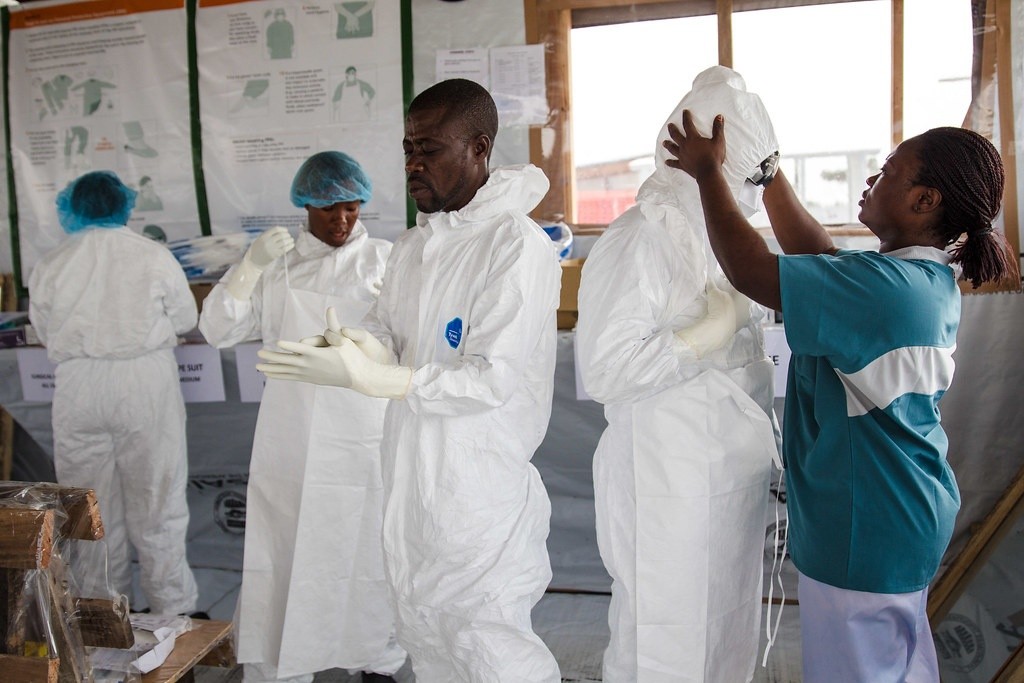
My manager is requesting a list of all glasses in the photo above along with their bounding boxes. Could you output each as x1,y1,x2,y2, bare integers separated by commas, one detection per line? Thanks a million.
747,150,780,187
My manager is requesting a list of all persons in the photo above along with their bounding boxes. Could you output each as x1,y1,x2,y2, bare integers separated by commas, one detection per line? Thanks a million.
577,65,780,683
662,108,1020,683
256,79,562,683
199,151,408,683
28,169,199,616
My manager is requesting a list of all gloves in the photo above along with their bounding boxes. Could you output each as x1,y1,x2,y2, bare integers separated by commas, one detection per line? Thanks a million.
225,227,295,301
677,276,752,359
256,307,413,399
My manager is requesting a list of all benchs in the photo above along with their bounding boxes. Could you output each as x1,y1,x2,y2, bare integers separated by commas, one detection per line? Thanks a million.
0,481,238,683
